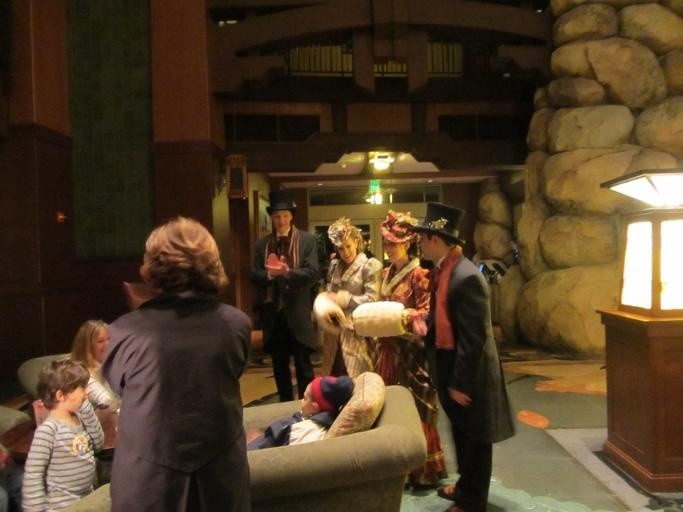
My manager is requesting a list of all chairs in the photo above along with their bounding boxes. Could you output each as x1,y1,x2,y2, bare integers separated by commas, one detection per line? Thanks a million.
16,354,72,400
55,482,111,512
238,384,428,512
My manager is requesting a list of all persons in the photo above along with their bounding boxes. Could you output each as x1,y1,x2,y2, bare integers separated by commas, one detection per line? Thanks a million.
250,188,319,402
21,359,105,511
413,202,515,511
99,216,252,510
375,210,448,490
320,216,383,380
246,376,354,452
69,319,118,407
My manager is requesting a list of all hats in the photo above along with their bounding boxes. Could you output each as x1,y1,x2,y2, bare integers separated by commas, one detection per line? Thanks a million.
412,201,465,244
379,210,416,243
328,217,350,246
266,190,297,213
311,376,354,410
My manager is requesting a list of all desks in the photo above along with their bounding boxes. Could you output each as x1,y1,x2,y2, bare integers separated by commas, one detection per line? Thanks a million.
594,301,682,492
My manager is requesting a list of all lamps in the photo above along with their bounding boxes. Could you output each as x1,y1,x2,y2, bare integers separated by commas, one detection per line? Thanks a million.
599,169,682,316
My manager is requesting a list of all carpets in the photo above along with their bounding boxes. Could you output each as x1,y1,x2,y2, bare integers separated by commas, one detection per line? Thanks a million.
238,366,301,404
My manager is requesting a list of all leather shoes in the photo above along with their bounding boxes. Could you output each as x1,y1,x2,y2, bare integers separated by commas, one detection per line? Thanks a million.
437,485,455,500
